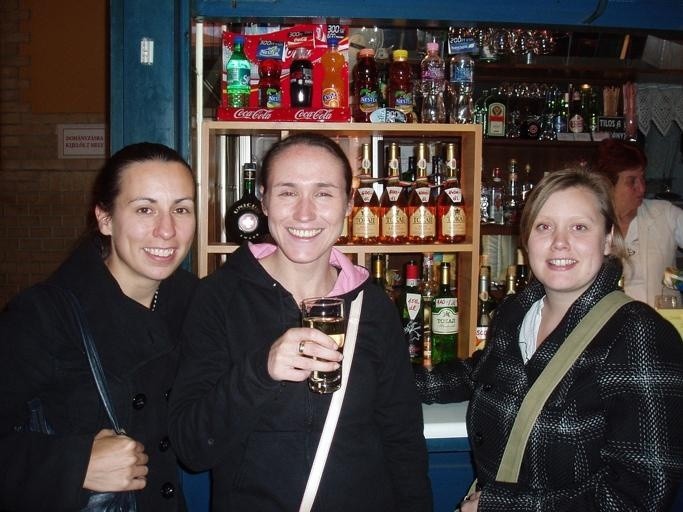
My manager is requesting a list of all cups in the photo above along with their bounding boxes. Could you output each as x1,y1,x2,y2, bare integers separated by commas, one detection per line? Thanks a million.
299,295,349,395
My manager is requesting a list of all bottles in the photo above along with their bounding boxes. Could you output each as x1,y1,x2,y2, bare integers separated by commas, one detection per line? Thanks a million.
519,164,534,218
320,37,344,108
225,36,250,108
516,249,527,292
488,167,504,225
472,81,600,141
449,52,473,124
402,155,416,201
479,168,489,224
257,59,282,108
504,159,519,226
408,143,436,244
426,155,444,198
385,49,414,123
333,203,349,245
401,259,423,365
475,266,495,354
420,42,445,123
224,162,267,246
617,275,625,294
350,143,379,244
503,264,517,300
436,142,467,244
379,143,407,245
420,256,434,361
543,171,549,177
430,262,458,368
289,45,313,108
352,49,380,122
371,254,385,291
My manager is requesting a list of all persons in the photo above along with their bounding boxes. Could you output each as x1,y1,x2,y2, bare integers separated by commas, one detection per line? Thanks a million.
164,134,438,510
584,136,683,311
411,164,680,510
1,141,201,512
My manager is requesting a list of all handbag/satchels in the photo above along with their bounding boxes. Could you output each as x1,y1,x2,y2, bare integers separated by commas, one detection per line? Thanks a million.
295,290,366,511
462,289,638,505
24,276,140,512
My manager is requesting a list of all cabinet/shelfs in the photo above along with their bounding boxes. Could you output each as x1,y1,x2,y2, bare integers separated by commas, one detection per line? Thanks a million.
477,236,534,348
208,251,472,368
473,76,623,138
482,145,598,223
209,135,474,245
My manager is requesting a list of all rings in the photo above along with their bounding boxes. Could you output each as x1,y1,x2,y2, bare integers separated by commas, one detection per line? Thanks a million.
297,339,307,353
